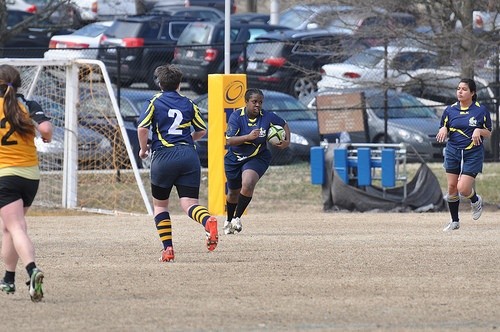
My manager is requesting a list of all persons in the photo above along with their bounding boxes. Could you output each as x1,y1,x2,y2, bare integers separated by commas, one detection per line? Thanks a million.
137,65,219,262
224,89,290,235
435,79,491,230
0,65,53,303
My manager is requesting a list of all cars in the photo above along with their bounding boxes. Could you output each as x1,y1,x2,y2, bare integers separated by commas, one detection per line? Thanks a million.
0,0,500,169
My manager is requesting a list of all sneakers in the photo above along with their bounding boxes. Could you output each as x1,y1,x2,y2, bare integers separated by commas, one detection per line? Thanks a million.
0,280,15,295
232,217,242,232
205,216,219,252
158,246,175,262
223,221,235,234
26,268,44,302
443,220,460,232
471,195,482,220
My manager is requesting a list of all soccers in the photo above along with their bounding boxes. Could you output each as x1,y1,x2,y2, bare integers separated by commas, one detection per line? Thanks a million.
267,124,286,144
